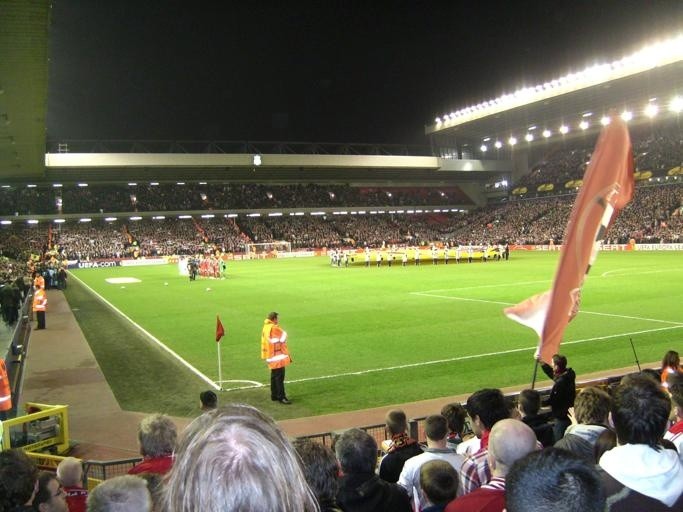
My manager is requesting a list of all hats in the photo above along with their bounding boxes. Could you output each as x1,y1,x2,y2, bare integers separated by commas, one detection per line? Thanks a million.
199,390,217,407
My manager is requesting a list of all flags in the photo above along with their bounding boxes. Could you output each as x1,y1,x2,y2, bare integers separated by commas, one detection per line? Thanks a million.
504,110,634,366
216,319,225,342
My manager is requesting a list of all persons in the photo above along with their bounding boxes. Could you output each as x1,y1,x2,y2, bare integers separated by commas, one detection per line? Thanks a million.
443,119,683,247
3,354,683,512
261,312,293,406
1,181,511,280
1,271,48,331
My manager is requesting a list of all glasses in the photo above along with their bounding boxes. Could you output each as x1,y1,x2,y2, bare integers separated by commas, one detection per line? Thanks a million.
51,485,64,499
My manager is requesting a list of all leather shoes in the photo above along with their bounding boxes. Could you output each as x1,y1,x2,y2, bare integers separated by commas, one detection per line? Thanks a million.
282,398,291,404
